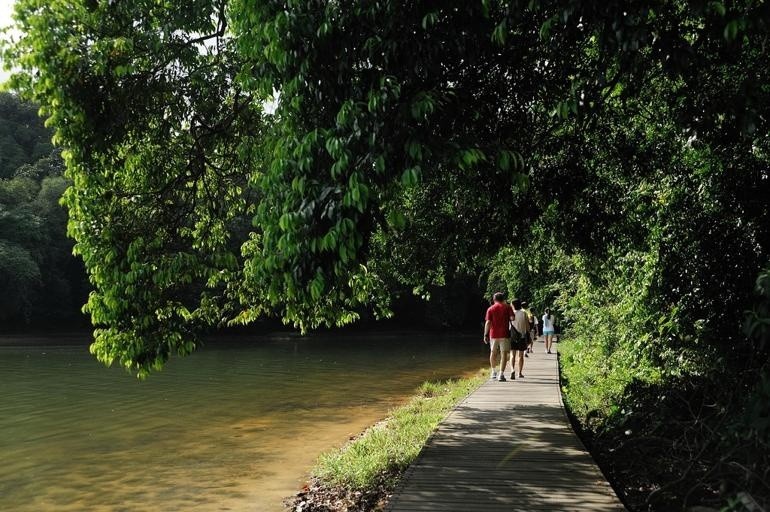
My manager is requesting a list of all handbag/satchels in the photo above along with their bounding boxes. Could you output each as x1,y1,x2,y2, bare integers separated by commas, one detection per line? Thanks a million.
509,325,521,343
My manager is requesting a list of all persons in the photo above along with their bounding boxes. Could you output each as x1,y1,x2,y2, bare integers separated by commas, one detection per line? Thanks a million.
520,301,533,358
542,307,556,354
527,307,539,353
508,298,528,379
483,292,515,381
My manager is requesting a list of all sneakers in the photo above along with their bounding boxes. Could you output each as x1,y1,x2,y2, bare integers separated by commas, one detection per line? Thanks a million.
492,371,524,381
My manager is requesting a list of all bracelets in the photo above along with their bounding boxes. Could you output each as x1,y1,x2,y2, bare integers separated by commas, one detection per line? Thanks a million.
484,335,488,337
